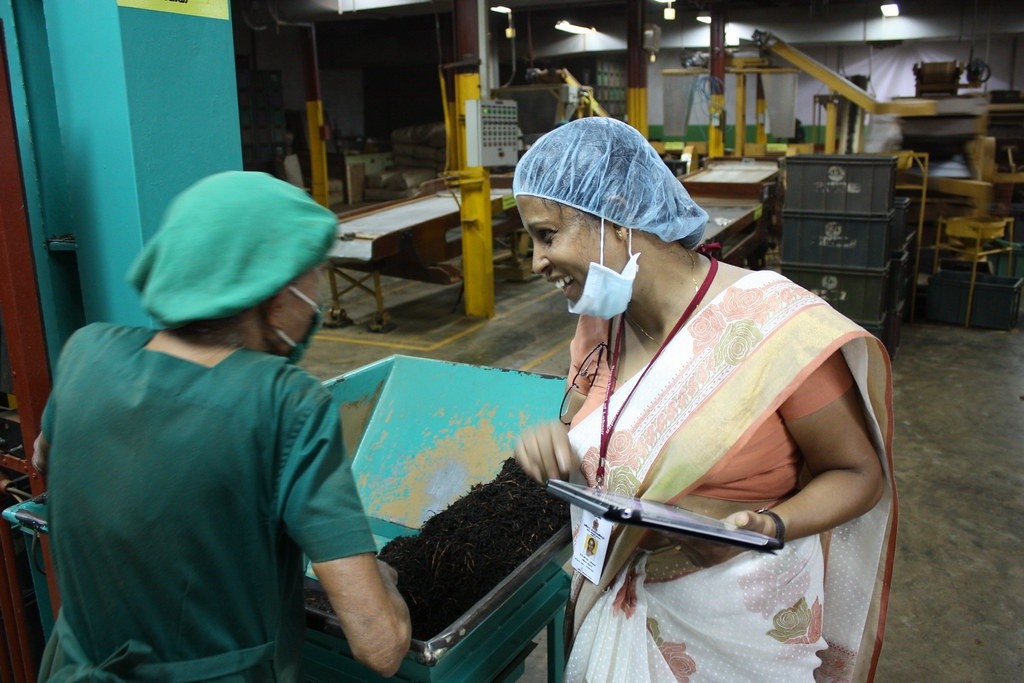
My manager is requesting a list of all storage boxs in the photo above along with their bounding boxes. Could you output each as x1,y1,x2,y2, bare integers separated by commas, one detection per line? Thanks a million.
780,153,1024,363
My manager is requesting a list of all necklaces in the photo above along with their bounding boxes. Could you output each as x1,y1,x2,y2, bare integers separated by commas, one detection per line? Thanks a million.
625,248,698,347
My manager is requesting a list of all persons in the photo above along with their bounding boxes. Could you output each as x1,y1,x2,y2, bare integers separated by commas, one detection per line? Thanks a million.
32,171,412,682
586,539,594,559
513,116,899,683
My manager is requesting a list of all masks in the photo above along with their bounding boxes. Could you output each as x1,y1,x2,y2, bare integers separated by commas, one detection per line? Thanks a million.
272,286,325,365
568,218,641,320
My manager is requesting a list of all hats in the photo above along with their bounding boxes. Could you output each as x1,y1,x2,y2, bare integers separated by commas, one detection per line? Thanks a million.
126,171,338,329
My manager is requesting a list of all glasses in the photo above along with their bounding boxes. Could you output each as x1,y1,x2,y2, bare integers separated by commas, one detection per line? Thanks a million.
559,341,612,426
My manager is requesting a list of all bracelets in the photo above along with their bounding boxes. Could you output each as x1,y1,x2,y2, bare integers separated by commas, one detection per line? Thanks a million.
755,507,785,555
